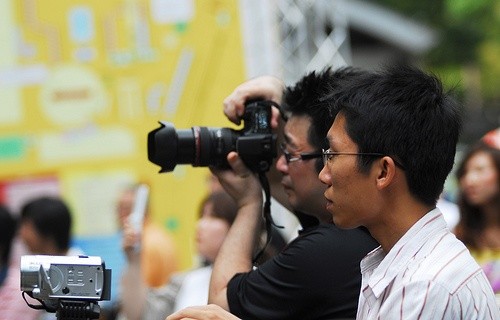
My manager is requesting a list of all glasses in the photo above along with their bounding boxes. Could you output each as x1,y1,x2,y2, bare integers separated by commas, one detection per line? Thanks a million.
322,148,405,169
280,141,323,165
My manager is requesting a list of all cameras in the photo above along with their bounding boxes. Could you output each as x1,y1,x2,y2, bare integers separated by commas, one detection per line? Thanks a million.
20,255,111,320
147,100,277,174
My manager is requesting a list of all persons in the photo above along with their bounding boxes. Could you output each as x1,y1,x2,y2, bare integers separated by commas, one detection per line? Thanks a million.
0,173,287,320
451,144,500,294
165,65,500,320
206,64,379,320
